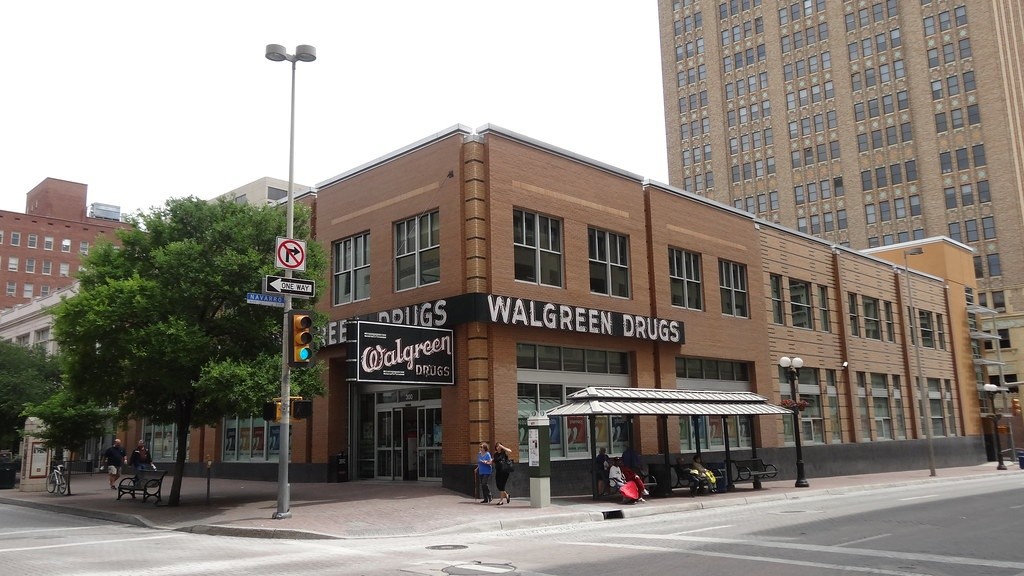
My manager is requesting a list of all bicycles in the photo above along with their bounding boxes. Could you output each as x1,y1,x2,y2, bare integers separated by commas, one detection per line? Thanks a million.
46,463,67,494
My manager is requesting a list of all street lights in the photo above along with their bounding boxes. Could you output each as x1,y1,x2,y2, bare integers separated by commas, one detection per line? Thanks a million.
264,43,316,519
779,357,809,487
984,384,1008,470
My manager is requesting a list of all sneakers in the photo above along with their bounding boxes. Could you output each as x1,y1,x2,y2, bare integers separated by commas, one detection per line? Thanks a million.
641,488,649,495
638,497,645,502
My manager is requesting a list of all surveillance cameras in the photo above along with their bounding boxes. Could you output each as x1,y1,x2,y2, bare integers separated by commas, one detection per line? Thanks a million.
842,362,848,367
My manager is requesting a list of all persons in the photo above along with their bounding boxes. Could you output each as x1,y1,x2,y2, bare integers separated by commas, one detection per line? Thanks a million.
129,440,153,487
474,441,512,505
101,439,128,490
420,428,432,476
676,455,717,497
596,448,649,502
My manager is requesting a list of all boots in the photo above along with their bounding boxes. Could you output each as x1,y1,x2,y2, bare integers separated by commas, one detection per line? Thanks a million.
480,492,492,503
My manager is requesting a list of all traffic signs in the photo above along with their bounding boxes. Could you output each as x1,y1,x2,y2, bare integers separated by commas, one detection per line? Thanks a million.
266,275,315,297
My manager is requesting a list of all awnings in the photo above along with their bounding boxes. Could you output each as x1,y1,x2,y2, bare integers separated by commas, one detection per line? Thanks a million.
547,386,794,501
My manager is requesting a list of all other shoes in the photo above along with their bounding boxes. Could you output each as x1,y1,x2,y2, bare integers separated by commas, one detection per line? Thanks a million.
710,488,719,494
111,485,117,490
507,495,510,503
497,502,504,505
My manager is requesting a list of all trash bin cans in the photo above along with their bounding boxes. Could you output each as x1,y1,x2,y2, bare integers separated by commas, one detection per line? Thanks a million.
0,467,16,489
647,463,671,497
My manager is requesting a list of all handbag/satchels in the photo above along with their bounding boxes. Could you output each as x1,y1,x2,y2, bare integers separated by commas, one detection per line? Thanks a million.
705,469,716,483
499,450,515,472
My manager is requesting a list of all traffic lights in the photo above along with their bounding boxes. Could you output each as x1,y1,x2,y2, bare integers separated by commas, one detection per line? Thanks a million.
290,400,312,419
263,401,281,422
288,309,315,367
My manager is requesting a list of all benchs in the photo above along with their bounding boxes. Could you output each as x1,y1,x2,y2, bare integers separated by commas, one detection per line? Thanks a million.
116,469,168,503
598,458,779,499
